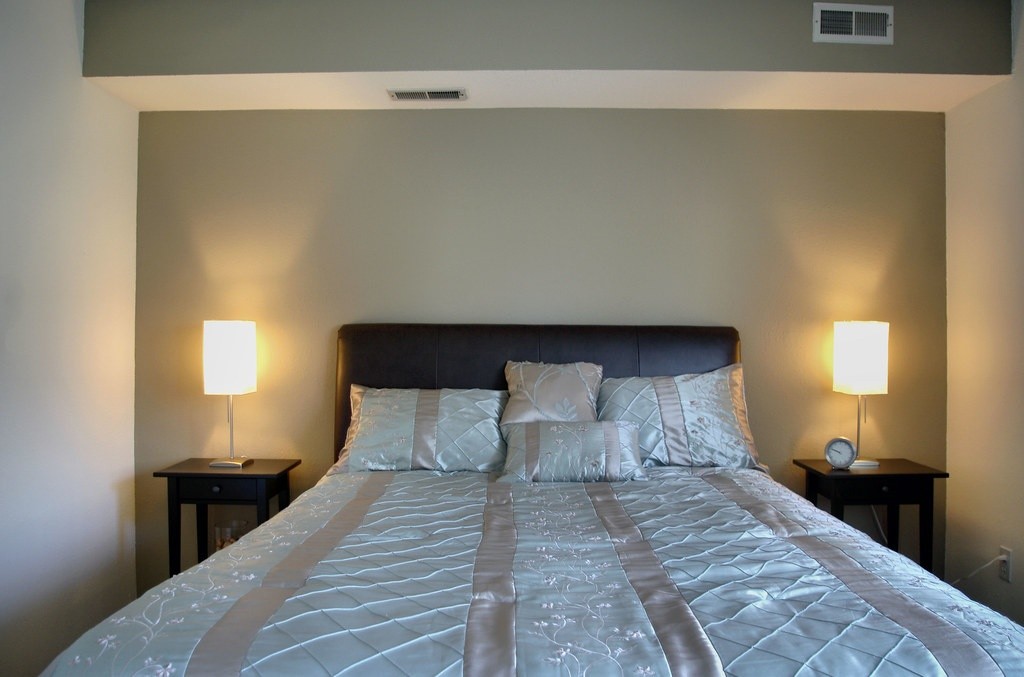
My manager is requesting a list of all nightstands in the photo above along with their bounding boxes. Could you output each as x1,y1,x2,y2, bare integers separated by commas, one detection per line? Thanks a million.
793,457,949,571
152,458,301,579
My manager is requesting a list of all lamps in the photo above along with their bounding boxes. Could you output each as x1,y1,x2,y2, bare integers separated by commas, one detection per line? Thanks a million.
203,320,258,468
832,321,889,467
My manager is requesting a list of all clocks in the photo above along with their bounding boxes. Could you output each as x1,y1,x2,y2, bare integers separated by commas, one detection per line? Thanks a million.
824,437,857,471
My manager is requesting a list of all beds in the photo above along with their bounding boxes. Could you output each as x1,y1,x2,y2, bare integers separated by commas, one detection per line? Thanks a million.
36,322,1024,677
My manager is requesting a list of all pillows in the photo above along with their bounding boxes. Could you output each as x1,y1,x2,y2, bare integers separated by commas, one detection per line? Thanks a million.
324,383,508,476
497,420,649,482
597,363,770,473
499,359,605,425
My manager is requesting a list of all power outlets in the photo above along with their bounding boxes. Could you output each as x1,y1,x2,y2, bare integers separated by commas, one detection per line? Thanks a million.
998,545,1012,583
